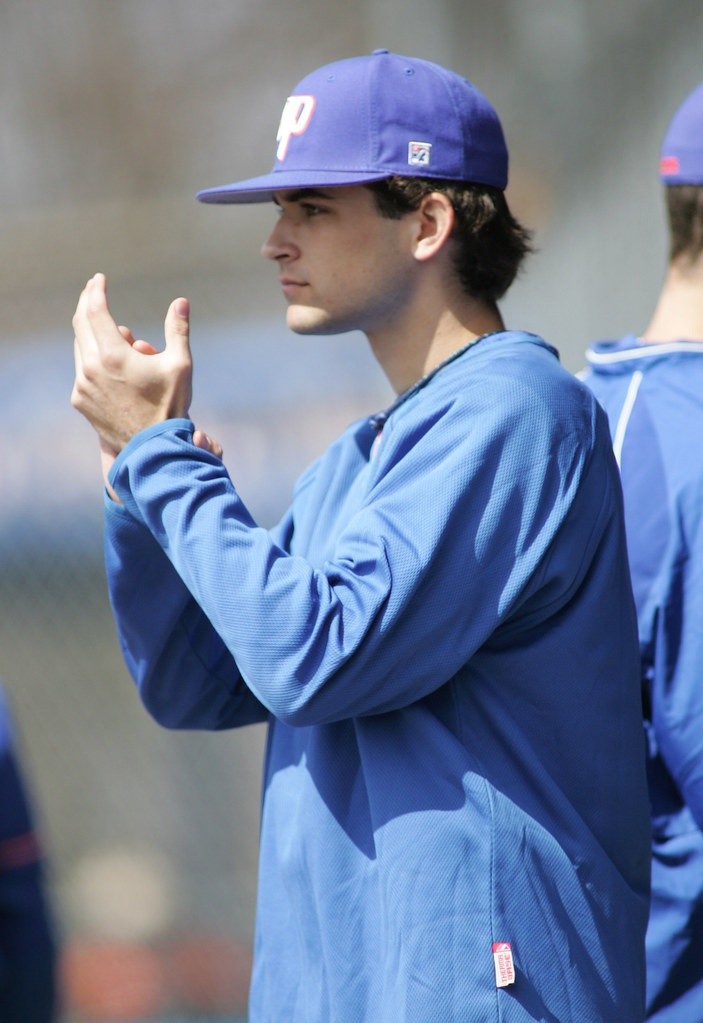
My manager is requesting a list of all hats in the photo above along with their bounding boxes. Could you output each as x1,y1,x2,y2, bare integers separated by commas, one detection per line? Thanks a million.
659,86,703,184
194,48,510,205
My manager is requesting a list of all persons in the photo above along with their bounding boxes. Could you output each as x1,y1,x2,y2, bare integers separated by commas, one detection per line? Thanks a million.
66,45,650,1023
565,82,703,1020
